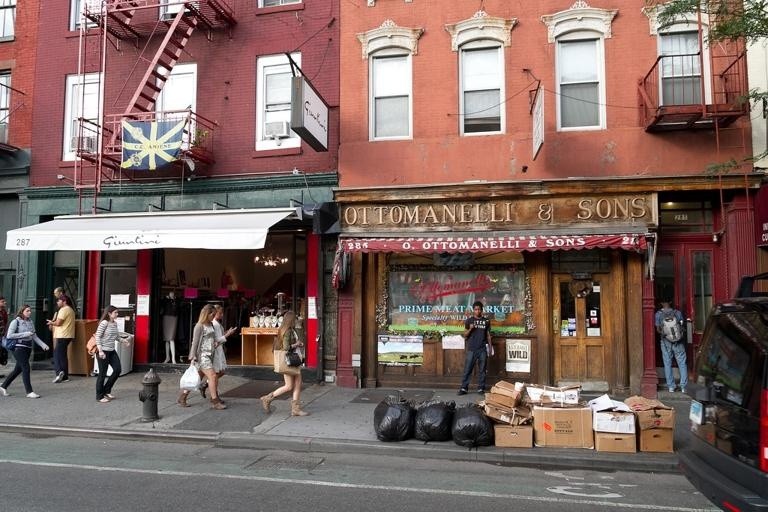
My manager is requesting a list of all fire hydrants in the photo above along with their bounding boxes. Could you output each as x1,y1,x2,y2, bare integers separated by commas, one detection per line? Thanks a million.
138,367,163,422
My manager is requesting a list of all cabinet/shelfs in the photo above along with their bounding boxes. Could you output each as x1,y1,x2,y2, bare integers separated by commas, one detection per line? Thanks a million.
240,327,281,367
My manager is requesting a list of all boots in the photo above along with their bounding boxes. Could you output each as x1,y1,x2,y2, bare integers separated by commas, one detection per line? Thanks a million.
210,390,226,409
291,399,309,415
260,392,275,412
178,393,190,407
199,382,208,398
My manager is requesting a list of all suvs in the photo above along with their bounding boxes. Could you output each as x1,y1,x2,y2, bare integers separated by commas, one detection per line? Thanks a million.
677,271,768,512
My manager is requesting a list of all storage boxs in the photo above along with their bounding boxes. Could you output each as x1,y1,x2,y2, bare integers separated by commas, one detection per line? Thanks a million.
485,380,676,454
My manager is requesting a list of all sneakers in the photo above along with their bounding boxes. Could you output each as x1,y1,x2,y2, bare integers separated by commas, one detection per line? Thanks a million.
26,391,40,398
0,382,11,396
669,387,674,392
478,389,485,394
681,389,687,393
456,389,467,395
53,371,71,382
98,397,110,402
105,393,115,399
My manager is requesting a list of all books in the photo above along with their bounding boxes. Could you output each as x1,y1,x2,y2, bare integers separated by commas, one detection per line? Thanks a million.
486,343,495,357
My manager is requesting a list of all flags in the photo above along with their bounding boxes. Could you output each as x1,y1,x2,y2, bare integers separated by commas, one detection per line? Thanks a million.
121,118,186,171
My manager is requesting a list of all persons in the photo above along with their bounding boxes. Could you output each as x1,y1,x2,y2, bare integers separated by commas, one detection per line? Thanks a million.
46,286,75,383
177,302,239,410
161,291,177,365
0,295,10,378
456,299,495,395
655,297,690,394
259,309,310,417
0,303,49,399
86,304,130,402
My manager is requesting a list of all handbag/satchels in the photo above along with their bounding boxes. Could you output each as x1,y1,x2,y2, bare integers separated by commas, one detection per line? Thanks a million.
180,359,201,391
86,334,100,354
0,346,8,365
273,342,303,375
1,319,19,349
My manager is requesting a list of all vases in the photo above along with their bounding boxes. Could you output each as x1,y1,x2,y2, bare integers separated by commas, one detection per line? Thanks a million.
192,147,209,162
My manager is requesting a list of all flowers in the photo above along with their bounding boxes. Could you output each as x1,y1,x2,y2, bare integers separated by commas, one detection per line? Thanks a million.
194,129,209,145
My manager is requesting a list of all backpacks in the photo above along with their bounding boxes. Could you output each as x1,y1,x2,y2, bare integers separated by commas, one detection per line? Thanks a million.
658,309,685,343
